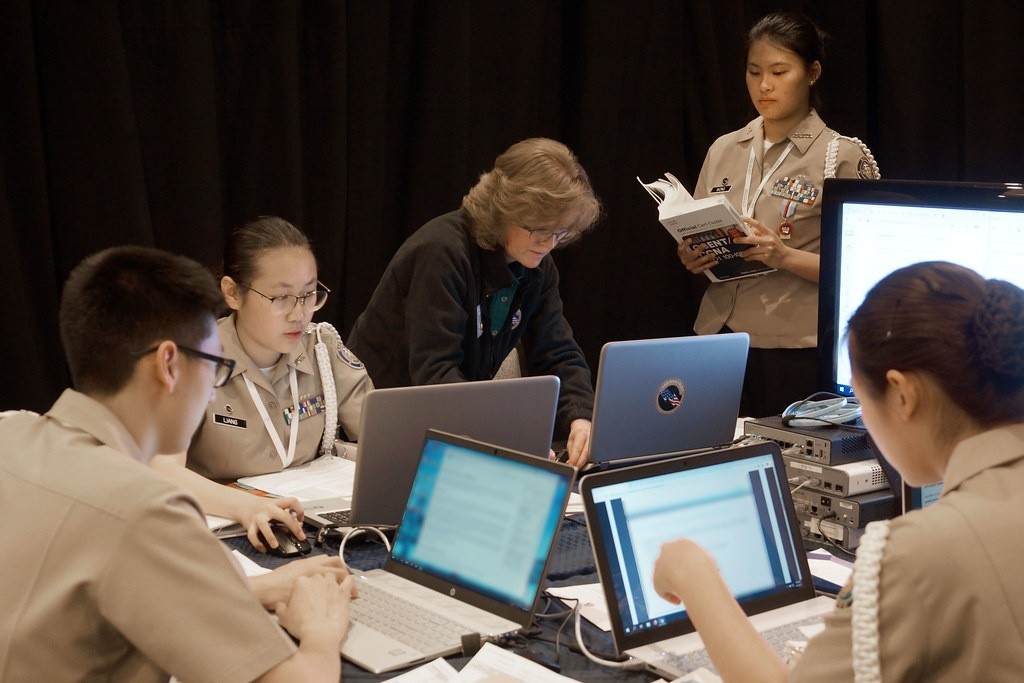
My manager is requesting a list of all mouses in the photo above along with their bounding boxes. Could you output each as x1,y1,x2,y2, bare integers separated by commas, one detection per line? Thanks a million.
261,526,308,558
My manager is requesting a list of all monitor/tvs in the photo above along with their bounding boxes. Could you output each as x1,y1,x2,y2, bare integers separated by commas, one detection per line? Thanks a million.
819,178,1024,403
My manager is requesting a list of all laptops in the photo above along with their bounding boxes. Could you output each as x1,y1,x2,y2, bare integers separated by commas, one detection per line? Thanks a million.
291,333,942,683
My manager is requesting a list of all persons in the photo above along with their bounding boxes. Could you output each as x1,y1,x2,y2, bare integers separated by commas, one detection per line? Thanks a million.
671,13,880,410
658,260,1022,683
183,211,380,551
345,137,607,470
0,250,358,683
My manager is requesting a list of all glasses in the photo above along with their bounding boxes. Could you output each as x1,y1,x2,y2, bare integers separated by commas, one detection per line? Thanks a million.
129,341,237,389
230,275,330,316
514,222,579,245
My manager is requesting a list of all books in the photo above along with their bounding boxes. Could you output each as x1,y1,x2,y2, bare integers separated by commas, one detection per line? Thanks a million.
636,171,779,284
